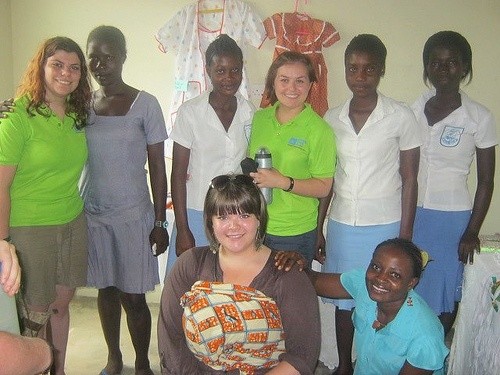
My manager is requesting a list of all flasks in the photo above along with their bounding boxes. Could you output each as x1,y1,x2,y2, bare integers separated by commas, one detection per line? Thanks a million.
254,148,272,203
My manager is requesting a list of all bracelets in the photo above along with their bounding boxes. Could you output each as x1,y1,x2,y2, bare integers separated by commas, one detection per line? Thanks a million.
282,177,294,192
3,236,13,244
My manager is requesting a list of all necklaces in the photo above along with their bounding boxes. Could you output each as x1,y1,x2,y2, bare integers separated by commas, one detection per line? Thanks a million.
371,306,394,329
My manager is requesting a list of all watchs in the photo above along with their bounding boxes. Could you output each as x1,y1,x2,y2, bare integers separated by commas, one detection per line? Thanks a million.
154,220,169,228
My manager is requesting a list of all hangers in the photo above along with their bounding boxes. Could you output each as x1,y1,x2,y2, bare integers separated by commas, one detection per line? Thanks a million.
180,0,251,14
272,0,333,26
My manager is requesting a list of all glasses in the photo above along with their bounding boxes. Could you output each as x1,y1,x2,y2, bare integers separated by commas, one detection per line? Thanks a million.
211,175,255,190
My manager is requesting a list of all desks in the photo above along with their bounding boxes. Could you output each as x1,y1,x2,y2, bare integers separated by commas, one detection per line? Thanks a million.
445,250,500,375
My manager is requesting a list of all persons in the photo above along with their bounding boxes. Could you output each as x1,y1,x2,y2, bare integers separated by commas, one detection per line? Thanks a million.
274,238,450,375
156,174,333,374
1,239,54,375
162,35,257,286
246,51,339,266
0,25,169,375
314,33,423,374
1,36,92,375
409,31,499,347
